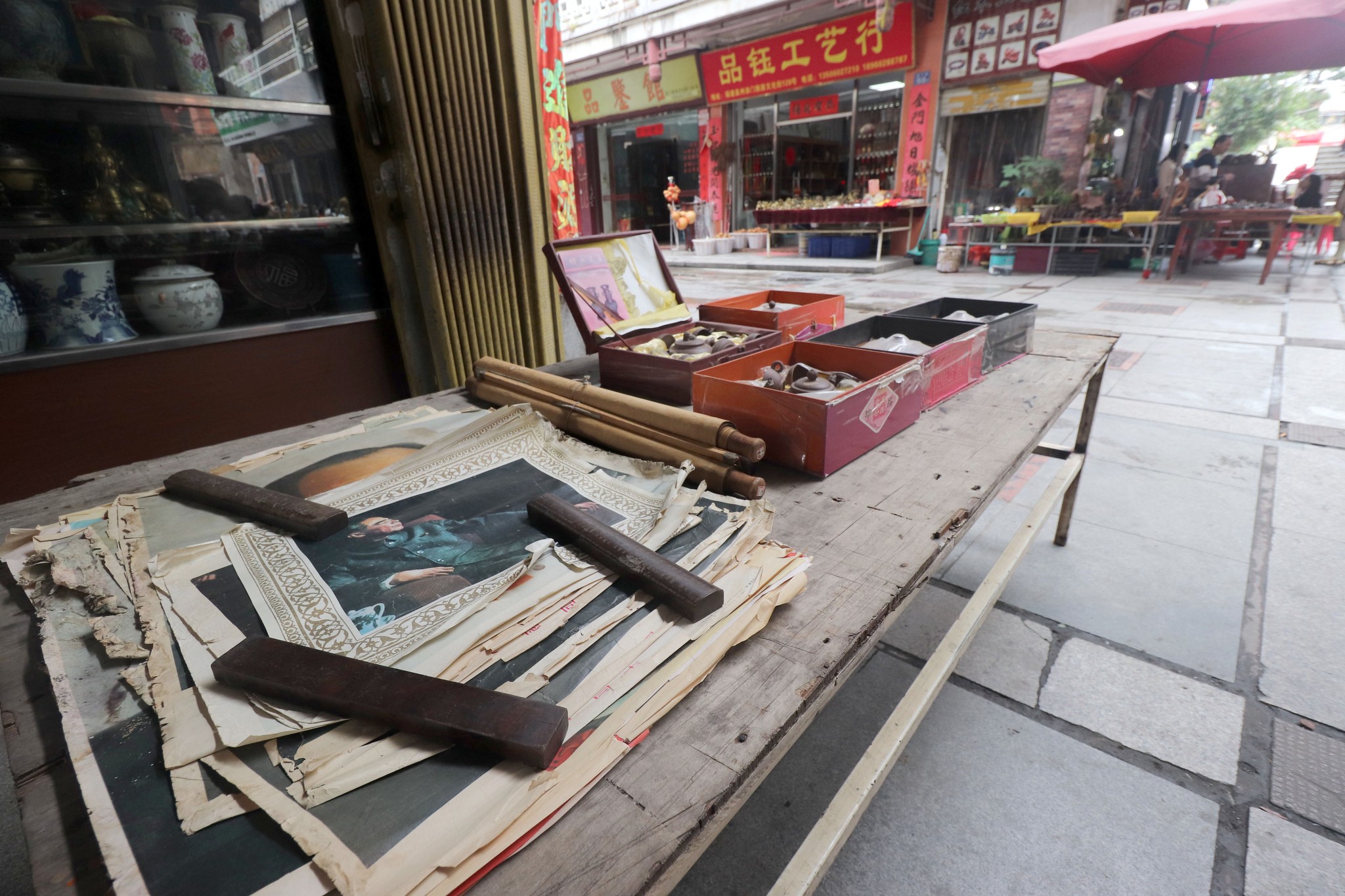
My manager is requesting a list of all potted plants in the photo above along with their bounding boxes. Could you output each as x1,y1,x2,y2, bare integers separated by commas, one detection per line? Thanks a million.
1004,154,1055,214
1033,187,1069,225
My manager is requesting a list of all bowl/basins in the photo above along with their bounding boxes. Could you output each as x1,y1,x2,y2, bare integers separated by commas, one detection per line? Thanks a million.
955,214,974,223
980,205,1042,224
691,232,767,256
1121,210,1161,224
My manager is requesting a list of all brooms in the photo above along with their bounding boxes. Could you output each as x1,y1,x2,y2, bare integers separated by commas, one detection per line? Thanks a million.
903,207,931,259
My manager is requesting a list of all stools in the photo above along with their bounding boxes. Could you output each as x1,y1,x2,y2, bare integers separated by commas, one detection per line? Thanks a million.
1222,229,1248,260
1214,219,1234,255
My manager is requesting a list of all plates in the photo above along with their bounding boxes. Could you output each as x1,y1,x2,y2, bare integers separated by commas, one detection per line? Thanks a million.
233,229,328,310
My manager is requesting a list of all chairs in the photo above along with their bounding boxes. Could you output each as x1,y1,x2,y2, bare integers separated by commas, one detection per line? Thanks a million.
1281,218,1333,256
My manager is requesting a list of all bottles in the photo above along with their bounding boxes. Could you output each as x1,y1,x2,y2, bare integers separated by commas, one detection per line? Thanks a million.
132,259,224,335
989,243,1016,276
0,274,30,356
0,0,263,98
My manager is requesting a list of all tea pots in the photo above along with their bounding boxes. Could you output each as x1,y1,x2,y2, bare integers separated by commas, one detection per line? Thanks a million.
788,369,837,394
667,331,716,356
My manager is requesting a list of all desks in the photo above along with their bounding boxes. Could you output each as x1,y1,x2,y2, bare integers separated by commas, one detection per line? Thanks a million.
749,189,922,269
954,201,1345,283
0,327,1128,896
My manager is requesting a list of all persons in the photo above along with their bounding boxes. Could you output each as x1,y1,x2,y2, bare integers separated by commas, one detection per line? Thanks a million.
326,501,599,608
1183,134,1232,207
1286,175,1323,237
265,442,425,500
1182,148,1209,170
1158,142,1189,234
1315,140,1345,266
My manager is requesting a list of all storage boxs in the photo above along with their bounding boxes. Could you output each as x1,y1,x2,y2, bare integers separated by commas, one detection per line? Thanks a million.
810,314,987,412
686,344,925,482
1012,242,1053,274
697,287,849,340
883,284,1044,375
543,225,782,407
1050,247,1092,276
808,234,874,261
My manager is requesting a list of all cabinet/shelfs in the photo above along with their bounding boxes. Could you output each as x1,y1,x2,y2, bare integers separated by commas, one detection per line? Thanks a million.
1,1,413,489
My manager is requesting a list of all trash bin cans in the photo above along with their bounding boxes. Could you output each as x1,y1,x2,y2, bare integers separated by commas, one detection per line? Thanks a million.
919,240,940,266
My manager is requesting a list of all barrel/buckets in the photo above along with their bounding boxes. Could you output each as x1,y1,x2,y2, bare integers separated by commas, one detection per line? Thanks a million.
936,246,964,273
920,239,941,265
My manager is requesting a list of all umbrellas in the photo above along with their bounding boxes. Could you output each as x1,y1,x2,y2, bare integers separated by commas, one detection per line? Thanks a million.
1034,0,1345,221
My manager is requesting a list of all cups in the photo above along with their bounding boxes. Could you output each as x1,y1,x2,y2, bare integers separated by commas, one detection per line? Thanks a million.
717,333,740,339
711,337,736,354
692,326,715,336
786,362,808,386
678,354,695,361
762,361,787,392
832,370,860,383
657,333,676,349
742,332,763,344
